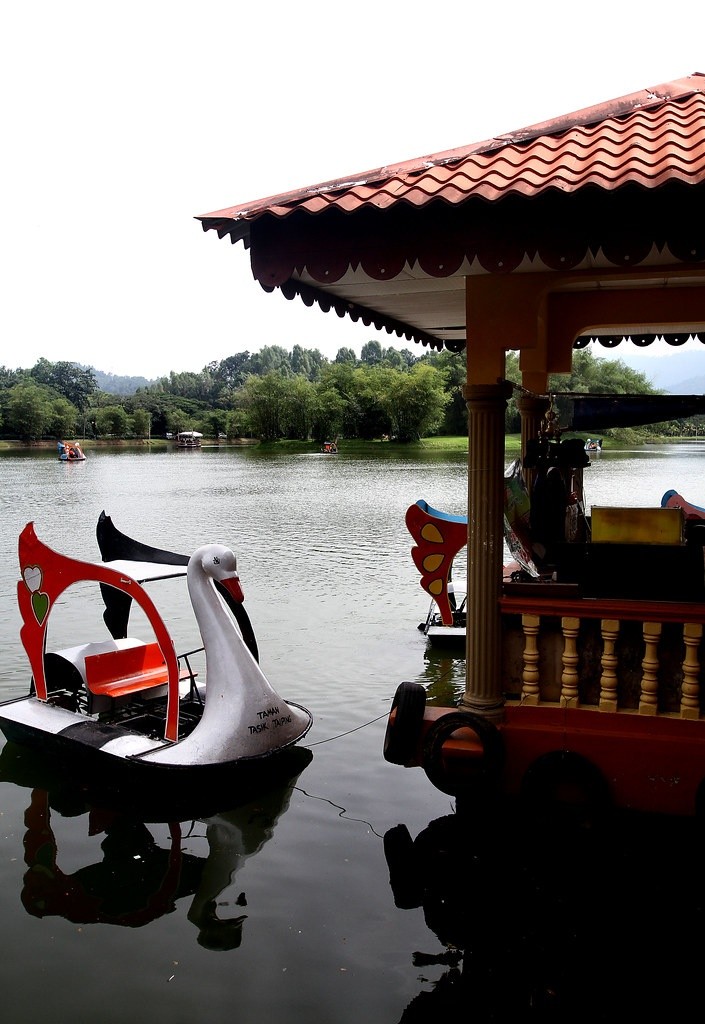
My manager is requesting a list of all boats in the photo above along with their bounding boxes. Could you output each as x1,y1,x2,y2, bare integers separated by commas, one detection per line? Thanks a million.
584,439,602,451
404,498,467,654
321,441,338,454
1,510,313,799
177,431,204,449
57,440,87,461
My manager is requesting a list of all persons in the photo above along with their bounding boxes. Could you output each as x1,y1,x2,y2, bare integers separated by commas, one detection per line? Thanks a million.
586,439,596,449
69,451,76,458
382,432,395,439
182,438,194,444
326,444,330,449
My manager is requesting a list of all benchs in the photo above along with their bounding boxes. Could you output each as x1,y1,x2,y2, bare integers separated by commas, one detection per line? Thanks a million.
85,640,198,725
